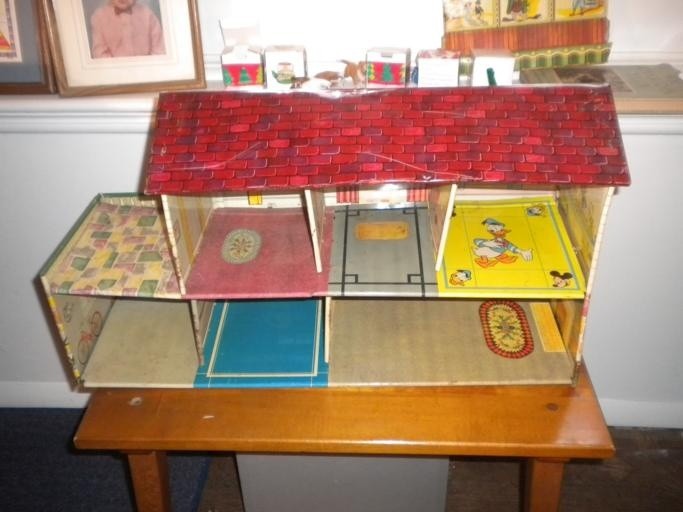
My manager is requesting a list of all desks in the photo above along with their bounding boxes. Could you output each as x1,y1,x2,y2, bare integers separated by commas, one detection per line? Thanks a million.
70,356,615,512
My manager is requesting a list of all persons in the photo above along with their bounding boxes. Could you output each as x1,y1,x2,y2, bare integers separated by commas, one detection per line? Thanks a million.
444,0,599,30
88,0,164,58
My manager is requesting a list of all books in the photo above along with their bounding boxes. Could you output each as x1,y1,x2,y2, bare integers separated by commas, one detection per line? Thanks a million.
520,61,681,115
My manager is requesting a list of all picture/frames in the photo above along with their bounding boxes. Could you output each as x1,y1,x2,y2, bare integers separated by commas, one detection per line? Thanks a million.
0,0,208,98
